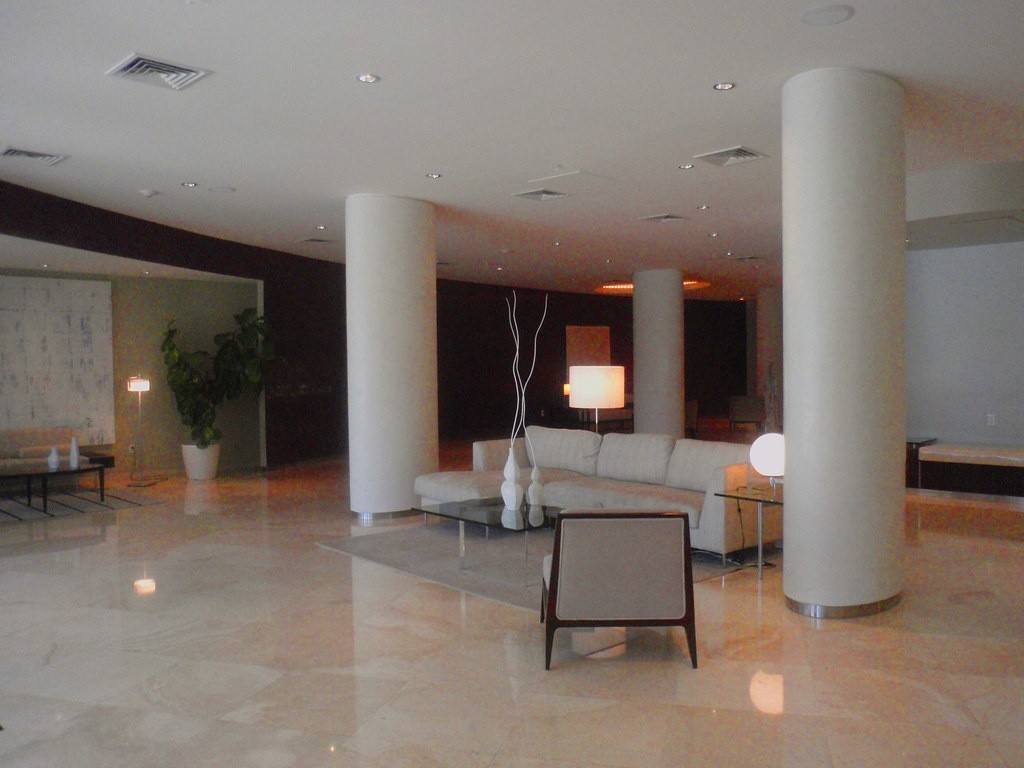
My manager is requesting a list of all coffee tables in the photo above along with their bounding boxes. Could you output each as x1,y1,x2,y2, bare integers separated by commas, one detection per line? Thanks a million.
409,494,567,589
714,484,784,579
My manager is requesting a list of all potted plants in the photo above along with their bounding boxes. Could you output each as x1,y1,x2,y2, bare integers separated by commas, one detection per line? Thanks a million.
161,306,290,479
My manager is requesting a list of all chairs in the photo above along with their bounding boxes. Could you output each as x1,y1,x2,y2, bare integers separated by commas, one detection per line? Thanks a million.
539,507,699,671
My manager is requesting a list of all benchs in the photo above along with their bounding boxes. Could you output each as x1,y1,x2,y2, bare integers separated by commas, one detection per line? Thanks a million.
917,442,1024,491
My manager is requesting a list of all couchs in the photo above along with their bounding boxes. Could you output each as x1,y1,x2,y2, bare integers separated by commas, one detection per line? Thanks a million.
415,424,784,569
0,427,115,491
127,373,157,488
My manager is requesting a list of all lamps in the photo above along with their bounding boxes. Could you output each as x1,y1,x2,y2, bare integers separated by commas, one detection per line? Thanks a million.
748,433,784,488
565,365,624,433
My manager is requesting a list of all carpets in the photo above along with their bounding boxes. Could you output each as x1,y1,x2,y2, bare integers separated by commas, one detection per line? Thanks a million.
317,517,784,613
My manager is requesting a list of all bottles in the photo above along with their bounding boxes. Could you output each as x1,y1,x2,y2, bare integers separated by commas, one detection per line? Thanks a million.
48,448,61,468
70,436,80,469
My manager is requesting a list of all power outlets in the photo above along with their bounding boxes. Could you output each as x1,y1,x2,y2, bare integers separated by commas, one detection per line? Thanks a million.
986,413,995,427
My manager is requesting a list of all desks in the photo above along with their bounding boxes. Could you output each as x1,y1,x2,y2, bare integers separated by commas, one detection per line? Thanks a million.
0,463,105,513
